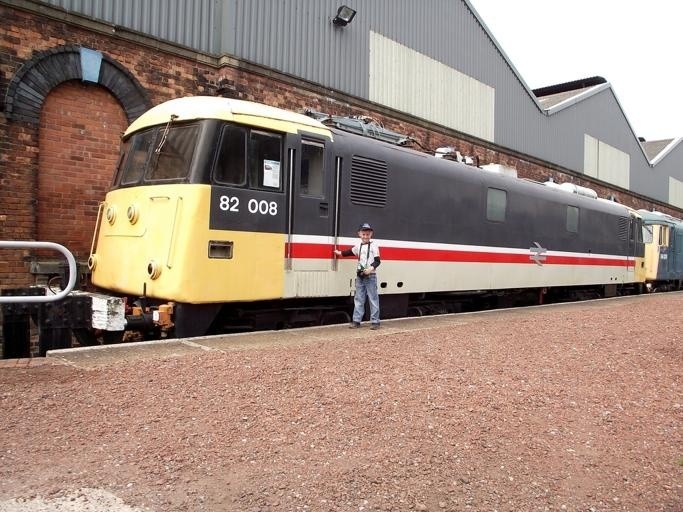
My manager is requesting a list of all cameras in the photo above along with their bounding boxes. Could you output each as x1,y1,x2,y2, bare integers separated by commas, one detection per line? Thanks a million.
357,264,365,272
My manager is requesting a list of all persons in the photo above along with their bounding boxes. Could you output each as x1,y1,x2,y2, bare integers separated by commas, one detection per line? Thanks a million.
333,221,381,330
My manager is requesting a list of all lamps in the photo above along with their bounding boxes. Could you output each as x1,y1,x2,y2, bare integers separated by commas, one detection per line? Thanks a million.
329,5,358,30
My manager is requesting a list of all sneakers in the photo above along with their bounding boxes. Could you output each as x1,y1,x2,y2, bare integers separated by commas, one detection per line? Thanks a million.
348,321,360,329
369,322,381,330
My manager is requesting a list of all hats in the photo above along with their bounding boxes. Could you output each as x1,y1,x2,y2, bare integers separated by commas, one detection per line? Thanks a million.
356,221,374,232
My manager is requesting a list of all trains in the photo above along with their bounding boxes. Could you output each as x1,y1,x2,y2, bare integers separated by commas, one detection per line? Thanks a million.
88,94,682,342
